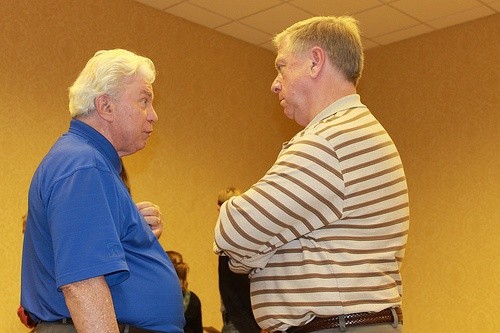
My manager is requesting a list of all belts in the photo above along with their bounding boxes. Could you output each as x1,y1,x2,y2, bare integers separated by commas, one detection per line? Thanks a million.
275,307,403,333
36,316,155,332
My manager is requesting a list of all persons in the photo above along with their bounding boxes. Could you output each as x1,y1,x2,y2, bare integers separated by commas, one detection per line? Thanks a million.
166,251,203,333
211,188,261,333
215,16,410,333
21,49,186,333
